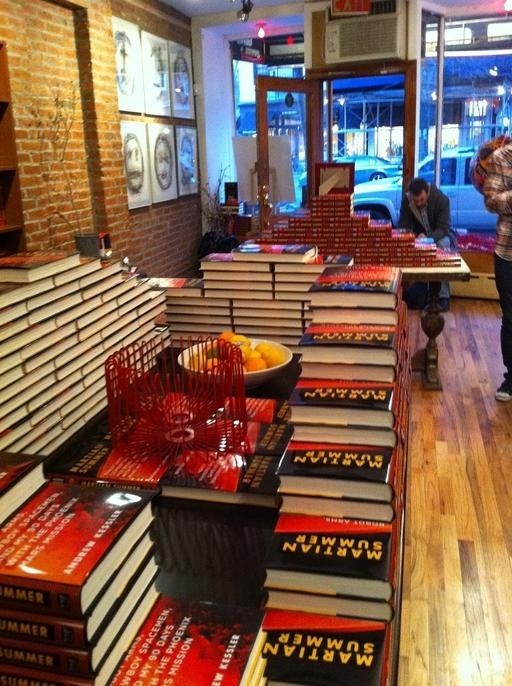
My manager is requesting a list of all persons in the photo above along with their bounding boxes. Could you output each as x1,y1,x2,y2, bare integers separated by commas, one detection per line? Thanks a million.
397,177,460,312
483,139,512,403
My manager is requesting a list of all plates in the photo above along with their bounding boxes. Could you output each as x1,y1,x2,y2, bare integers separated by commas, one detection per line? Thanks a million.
176,337,295,390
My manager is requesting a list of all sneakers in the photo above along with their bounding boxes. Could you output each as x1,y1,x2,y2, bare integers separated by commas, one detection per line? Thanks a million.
495,372,512,402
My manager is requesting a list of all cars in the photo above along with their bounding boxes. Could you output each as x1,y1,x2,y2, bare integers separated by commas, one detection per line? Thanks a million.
352,146,502,231
296,152,404,188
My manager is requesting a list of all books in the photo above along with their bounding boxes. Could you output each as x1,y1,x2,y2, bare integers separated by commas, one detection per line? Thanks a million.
1,193,462,686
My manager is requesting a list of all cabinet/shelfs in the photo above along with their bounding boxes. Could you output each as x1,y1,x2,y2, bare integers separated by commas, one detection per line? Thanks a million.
0,41,28,258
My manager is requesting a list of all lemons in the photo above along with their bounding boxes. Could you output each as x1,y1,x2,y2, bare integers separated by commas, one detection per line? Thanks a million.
189,331,287,374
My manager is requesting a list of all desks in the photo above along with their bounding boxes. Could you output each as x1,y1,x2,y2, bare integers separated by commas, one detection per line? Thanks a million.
399,253,471,390
